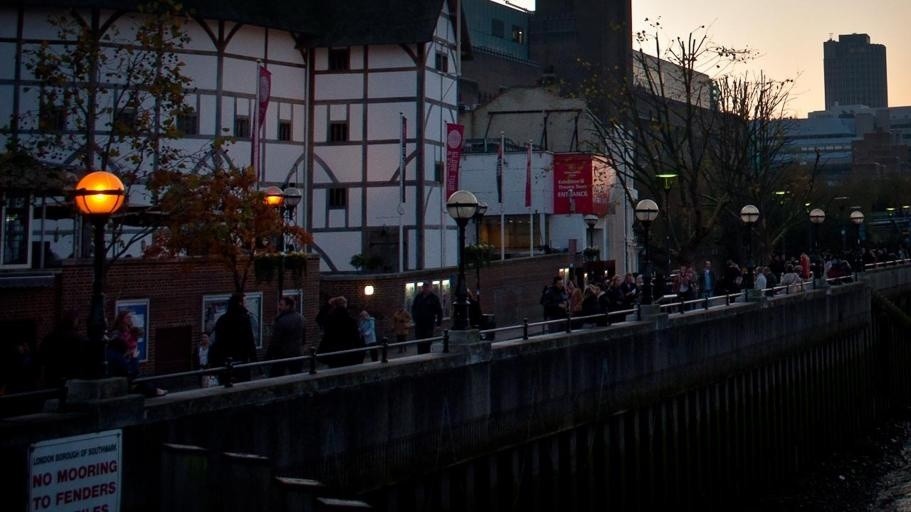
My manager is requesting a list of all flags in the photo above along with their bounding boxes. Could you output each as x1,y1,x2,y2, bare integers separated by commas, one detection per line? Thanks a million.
249,67,274,177
446,121,466,203
525,148,532,209
400,116,410,203
496,140,504,202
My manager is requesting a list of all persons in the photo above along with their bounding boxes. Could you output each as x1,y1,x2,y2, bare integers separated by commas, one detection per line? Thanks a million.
451,288,484,330
658,259,717,313
108,312,142,383
392,282,442,354
43,311,85,395
727,239,911,303
319,298,377,367
544,274,637,331
194,294,305,387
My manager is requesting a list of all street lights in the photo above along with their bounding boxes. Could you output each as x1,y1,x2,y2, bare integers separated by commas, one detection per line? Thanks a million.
446,189,477,331
66,167,125,381
846,207,868,266
809,204,827,280
634,199,661,307
739,204,760,291
264,186,301,256
584,210,598,252
